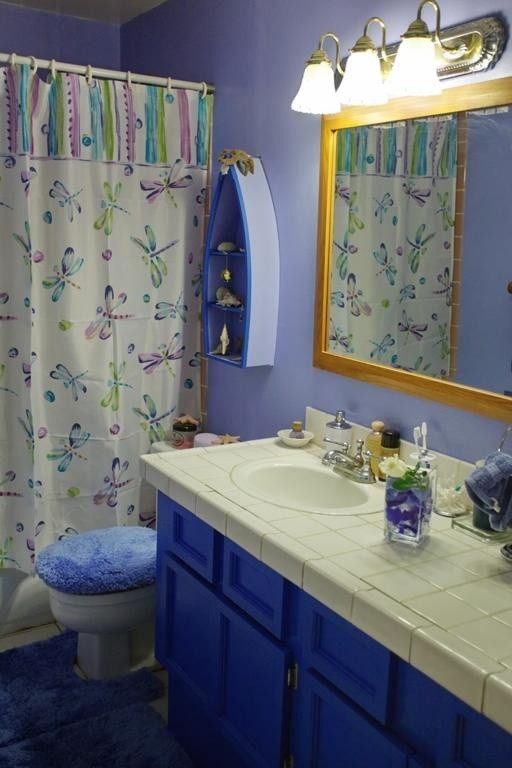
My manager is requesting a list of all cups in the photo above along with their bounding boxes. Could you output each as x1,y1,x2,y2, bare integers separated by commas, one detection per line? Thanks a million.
407,451,438,473
435,473,469,518
472,456,509,530
383,478,434,550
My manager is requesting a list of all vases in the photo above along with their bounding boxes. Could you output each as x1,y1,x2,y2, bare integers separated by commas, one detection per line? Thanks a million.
381,468,435,547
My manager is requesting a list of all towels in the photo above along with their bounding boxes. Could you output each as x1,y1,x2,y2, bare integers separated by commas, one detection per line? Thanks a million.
462,451,512,532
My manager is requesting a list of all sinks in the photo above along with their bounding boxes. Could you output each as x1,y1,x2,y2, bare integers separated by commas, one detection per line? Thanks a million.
231,454,385,515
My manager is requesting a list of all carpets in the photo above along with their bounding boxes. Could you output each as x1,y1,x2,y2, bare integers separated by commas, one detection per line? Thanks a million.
1,627,193,768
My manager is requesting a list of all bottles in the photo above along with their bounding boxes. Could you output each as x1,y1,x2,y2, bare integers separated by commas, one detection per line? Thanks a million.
288,421,304,439
379,429,401,481
366,419,387,480
174,422,217,451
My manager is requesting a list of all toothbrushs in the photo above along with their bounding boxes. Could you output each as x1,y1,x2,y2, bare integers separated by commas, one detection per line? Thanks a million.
413,425,424,458
421,421,427,453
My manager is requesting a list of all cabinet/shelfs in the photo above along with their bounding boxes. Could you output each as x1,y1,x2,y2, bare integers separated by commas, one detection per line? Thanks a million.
201,157,276,368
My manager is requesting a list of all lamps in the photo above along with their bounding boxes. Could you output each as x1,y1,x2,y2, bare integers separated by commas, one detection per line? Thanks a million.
291,0,511,115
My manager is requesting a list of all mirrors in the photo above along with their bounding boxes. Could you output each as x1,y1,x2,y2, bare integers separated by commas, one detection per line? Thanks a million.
311,77,511,420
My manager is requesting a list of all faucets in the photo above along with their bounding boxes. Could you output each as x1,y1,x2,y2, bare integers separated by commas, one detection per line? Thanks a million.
321,438,365,469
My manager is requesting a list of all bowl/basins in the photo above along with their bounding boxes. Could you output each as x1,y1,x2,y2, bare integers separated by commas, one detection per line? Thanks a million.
276,429,314,446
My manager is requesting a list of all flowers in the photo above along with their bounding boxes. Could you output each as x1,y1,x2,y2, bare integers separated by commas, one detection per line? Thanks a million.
379,450,428,533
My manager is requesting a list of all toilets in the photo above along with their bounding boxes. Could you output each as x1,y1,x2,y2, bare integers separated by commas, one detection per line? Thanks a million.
33,441,191,682
